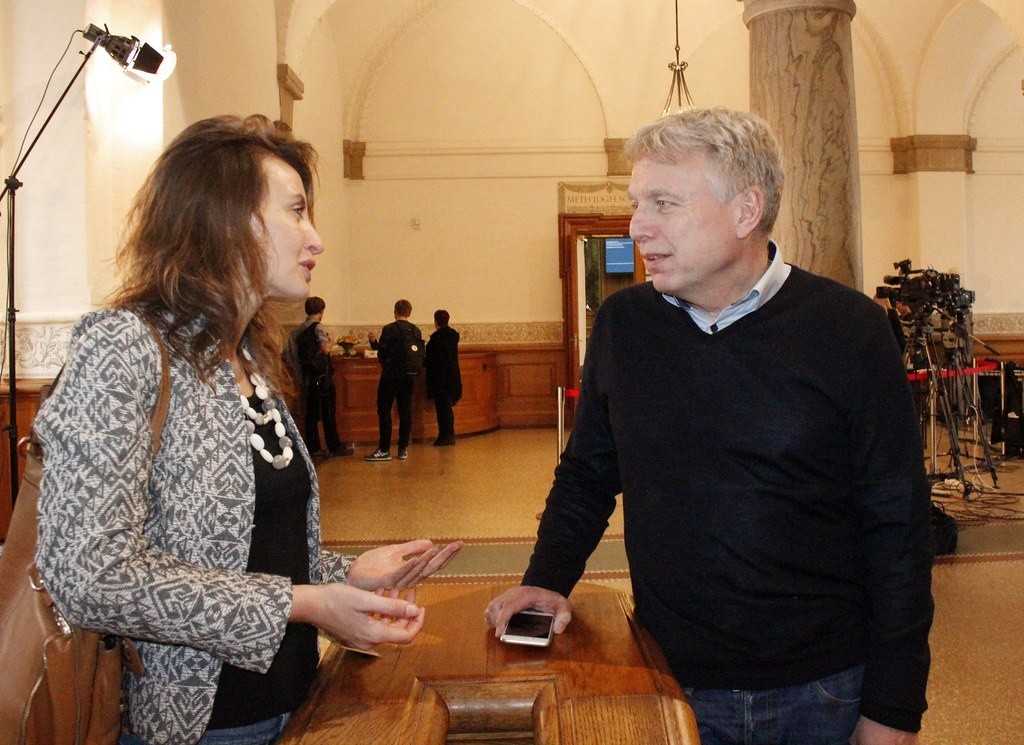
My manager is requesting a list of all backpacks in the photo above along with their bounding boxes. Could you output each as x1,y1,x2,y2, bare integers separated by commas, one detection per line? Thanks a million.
296,321,329,375
388,322,424,379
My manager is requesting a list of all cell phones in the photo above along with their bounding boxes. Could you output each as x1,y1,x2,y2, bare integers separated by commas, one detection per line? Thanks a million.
500,610,555,647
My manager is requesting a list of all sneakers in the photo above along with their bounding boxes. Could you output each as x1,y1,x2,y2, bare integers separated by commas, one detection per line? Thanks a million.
399,449,408,459
364,449,392,460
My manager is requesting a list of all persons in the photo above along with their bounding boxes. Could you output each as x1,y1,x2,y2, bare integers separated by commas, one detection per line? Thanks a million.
293,297,355,461
367,299,424,462
484,106,936,745
423,311,461,446
33,113,463,745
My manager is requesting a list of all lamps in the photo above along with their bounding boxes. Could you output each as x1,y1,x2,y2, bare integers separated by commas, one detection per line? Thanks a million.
657,0,696,119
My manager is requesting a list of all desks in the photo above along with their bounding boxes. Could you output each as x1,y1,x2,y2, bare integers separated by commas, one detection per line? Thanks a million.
280,582,700,745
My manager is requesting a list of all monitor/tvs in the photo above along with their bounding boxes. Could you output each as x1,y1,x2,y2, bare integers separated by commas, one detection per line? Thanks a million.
604,238,635,276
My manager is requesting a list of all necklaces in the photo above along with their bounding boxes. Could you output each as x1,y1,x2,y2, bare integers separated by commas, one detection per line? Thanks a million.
229,345,293,468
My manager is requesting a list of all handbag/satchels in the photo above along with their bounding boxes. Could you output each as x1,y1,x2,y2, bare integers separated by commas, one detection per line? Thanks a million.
0,305,169,745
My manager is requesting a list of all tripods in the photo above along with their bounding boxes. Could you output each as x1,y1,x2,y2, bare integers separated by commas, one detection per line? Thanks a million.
897,301,1001,498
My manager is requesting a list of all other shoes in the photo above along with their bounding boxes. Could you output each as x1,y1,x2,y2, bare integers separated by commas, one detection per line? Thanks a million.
433,437,455,445
310,449,332,459
328,445,353,456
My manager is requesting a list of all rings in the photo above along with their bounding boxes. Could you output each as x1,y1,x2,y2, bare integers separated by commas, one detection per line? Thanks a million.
419,574,428,580
